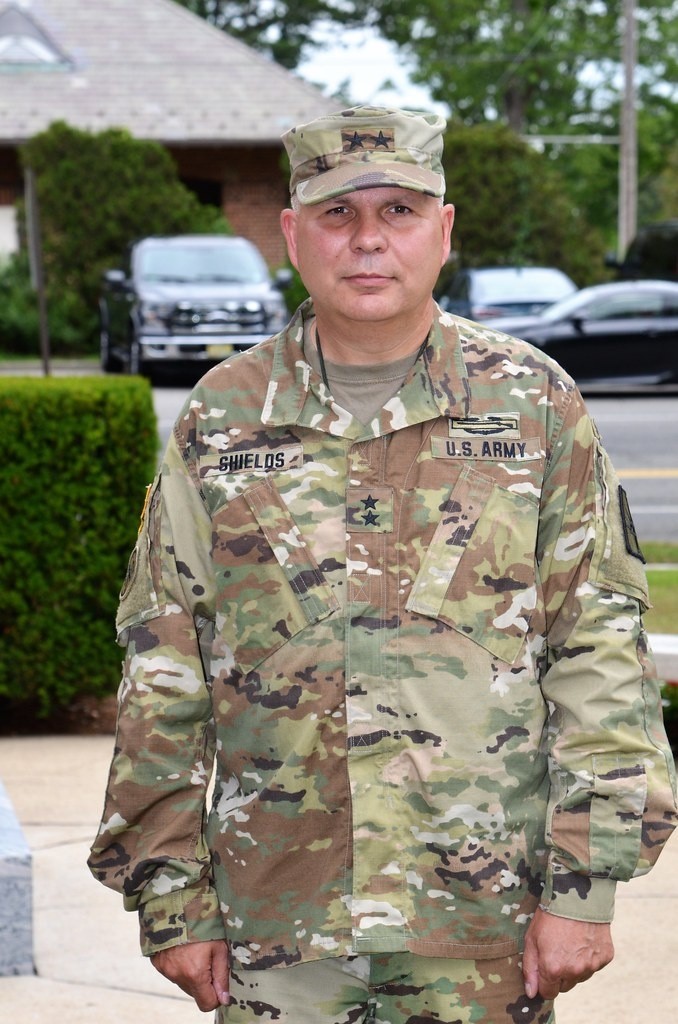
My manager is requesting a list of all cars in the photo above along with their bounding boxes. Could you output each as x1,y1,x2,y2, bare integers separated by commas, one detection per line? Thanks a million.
433,264,579,319
472,276,678,397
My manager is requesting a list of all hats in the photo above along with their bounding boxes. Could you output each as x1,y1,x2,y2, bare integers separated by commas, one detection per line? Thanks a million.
283,105,447,205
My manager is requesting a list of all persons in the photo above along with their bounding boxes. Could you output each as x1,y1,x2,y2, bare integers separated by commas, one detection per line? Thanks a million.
88,103,678,1023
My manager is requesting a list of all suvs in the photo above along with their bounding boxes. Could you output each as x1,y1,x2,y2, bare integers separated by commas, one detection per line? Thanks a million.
95,232,295,385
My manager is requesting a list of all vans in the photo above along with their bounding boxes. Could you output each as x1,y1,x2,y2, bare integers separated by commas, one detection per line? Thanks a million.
603,220,678,283
